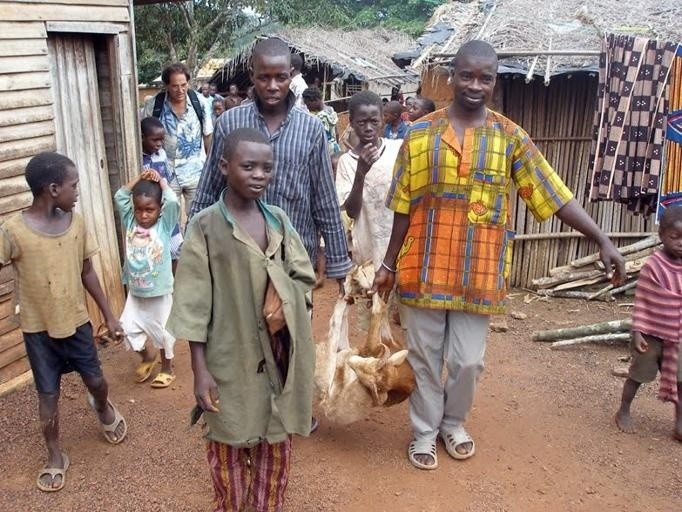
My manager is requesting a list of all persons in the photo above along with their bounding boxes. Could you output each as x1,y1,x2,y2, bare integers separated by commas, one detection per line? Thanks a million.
141,51,435,297
610,203,682,442
111,168,181,391
366,39,626,471
187,36,354,299
0,151,127,491
166,128,316,512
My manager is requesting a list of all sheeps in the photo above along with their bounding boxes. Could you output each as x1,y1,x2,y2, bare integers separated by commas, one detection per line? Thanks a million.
311,259,417,426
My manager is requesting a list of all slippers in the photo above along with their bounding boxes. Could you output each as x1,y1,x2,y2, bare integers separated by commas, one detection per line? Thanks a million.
133,350,159,384
439,422,477,462
406,434,439,471
84,386,128,446
149,370,177,389
35,452,72,494
